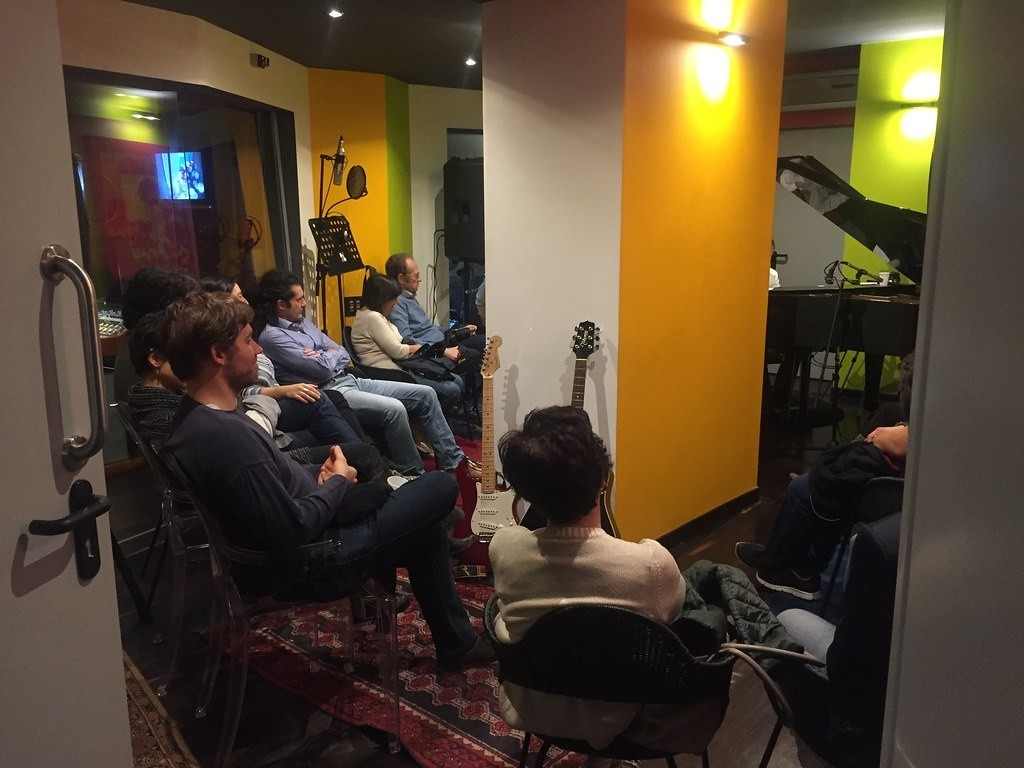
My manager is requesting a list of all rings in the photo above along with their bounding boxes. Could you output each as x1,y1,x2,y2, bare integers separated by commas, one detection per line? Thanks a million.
303,388,308,393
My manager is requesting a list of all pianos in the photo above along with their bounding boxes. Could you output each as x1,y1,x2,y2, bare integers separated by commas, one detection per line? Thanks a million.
765,155,927,427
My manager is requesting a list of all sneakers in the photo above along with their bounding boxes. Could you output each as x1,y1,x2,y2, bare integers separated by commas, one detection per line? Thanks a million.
755,567,823,601
734,541,788,570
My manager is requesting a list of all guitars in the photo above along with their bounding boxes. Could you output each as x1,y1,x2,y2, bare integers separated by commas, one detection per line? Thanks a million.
451,335,522,566
518,319,619,541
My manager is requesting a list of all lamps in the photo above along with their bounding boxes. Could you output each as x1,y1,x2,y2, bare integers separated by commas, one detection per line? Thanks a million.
900,102,938,110
718,31,749,45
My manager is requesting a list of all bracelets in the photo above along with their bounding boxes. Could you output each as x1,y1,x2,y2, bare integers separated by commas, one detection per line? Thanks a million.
895,422,908,428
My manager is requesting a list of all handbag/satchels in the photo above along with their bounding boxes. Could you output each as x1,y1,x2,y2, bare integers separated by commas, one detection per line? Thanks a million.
393,343,456,382
439,343,472,371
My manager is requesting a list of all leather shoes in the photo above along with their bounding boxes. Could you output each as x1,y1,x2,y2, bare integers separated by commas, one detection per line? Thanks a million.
446,534,478,552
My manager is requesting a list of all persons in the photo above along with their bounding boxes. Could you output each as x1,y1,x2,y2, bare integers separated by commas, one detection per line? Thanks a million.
762,239,798,412
737,427,908,768
120,253,486,619
162,290,499,671
488,404,686,749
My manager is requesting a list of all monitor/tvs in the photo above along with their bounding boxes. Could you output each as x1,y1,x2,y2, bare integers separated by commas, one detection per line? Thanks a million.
153,152,208,202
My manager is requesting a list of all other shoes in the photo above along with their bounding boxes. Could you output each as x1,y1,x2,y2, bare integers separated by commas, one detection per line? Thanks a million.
351,594,410,621
384,460,416,476
452,460,484,480
442,506,465,526
770,394,800,412
442,632,501,673
414,438,436,458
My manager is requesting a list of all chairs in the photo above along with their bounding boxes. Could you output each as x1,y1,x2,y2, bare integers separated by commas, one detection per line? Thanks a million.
109,326,906,768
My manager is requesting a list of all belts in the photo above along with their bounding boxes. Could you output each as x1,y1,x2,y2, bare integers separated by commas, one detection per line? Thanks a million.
318,369,346,390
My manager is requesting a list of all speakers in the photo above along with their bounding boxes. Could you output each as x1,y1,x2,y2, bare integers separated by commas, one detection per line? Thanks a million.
443,156,485,266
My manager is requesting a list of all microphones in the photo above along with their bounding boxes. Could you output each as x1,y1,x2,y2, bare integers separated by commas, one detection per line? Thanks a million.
824,261,840,284
333,135,345,185
